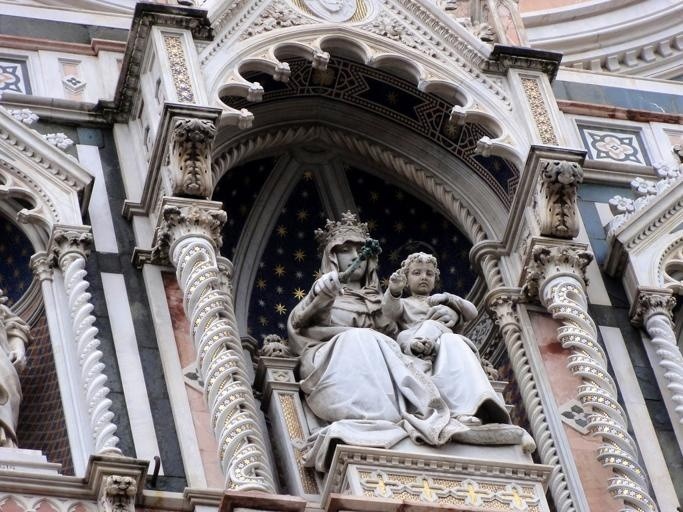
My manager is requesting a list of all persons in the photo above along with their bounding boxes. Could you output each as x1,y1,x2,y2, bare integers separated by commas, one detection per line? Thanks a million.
379,246,513,424
0,288,35,449
286,210,468,474
